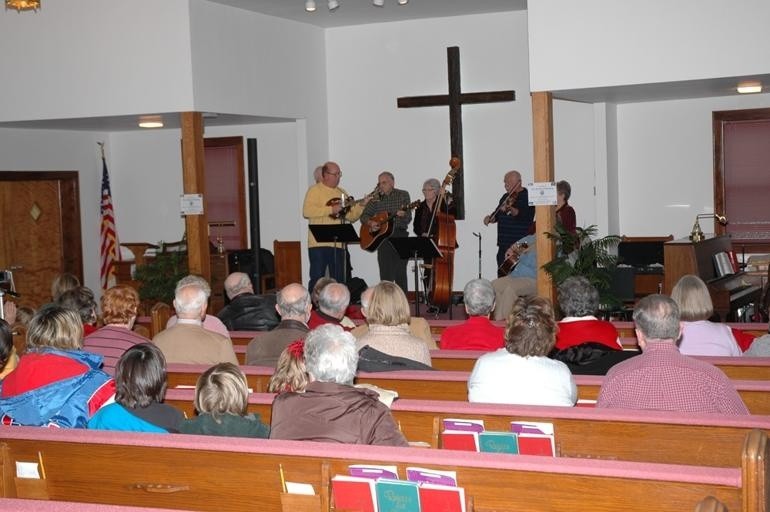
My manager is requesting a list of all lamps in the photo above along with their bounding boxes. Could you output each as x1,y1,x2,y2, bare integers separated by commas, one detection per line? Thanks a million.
305,0,408,15
3,0,42,13
689,209,729,242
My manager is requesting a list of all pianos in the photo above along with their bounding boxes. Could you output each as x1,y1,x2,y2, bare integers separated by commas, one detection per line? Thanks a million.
664,234,767,322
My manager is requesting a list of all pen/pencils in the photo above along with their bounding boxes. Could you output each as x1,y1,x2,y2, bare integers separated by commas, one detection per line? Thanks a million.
398,420,401,431
38,451,46,479
279,462,288,493
184,411,188,419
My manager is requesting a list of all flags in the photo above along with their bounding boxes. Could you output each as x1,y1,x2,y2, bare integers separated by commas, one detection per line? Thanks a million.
99,157,122,290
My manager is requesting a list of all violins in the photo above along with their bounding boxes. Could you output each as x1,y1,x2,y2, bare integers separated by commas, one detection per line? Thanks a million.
503,192,517,217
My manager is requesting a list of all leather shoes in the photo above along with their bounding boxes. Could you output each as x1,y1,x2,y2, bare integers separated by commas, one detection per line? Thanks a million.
426,306,439,313
438,305,449,314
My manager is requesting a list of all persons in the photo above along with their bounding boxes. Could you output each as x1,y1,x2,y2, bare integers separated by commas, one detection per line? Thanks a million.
484,171,535,278
491,214,563,321
313,166,353,280
553,275,623,349
413,179,459,313
670,275,742,357
744,282,768,357
303,161,374,294
468,296,578,407
596,295,750,416
528,180,579,249
179,363,270,438
3,306,116,429
439,278,506,353
1,276,439,392
269,323,410,444
360,172,412,293
87,341,185,433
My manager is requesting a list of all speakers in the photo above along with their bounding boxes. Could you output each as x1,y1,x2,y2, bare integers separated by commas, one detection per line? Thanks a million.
594,267,635,303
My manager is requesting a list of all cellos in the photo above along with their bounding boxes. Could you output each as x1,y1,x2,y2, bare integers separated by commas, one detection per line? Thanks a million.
423,158,462,310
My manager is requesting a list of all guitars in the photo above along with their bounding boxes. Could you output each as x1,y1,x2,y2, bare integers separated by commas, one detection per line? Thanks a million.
498,241,538,276
360,199,419,252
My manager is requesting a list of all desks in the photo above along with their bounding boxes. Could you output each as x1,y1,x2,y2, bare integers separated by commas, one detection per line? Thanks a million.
614,238,674,318
113,239,302,318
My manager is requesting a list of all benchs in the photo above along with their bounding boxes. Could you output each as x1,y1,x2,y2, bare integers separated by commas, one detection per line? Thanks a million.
0,319,769,511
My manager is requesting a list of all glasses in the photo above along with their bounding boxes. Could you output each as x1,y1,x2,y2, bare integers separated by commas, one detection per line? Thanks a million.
421,188,437,193
325,171,343,177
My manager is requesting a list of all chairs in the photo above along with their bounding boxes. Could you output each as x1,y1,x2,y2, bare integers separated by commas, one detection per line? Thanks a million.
599,266,637,321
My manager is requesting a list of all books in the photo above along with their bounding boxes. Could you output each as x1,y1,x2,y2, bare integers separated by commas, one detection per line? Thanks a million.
715,252,735,277
331,464,466,512
441,418,555,457
